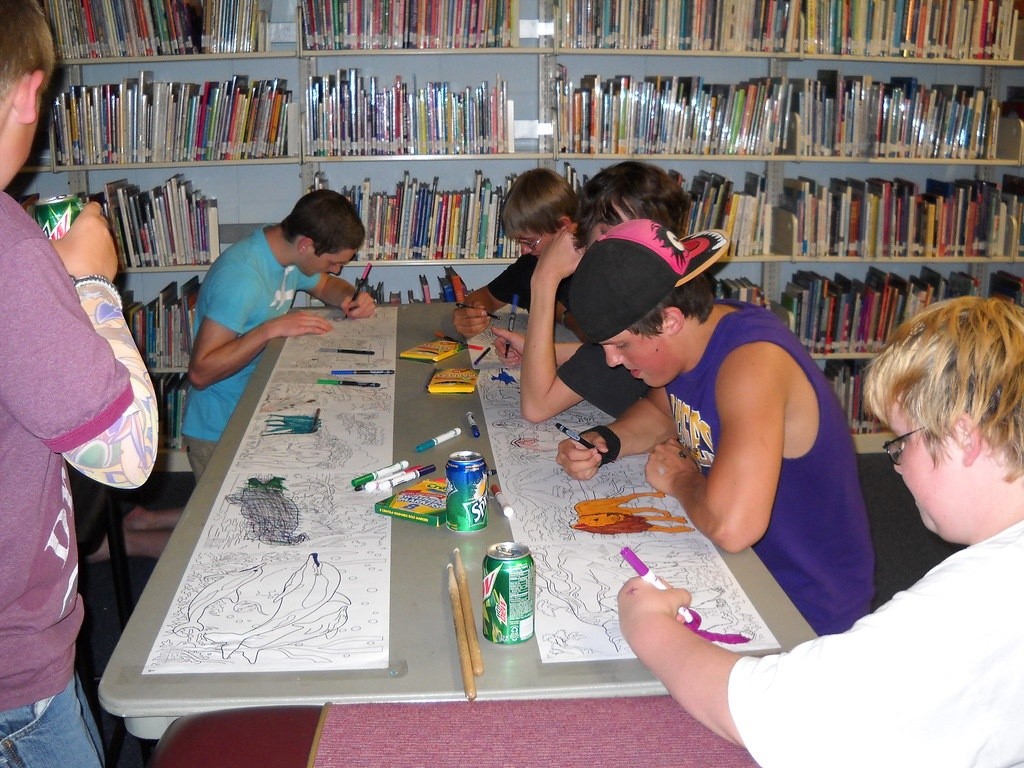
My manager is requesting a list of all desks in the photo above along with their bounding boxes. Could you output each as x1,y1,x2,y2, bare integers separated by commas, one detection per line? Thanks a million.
97,302,817,739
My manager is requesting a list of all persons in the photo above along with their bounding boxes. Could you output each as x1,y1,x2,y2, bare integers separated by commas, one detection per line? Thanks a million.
451,165,579,340
556,219,876,637
0,0,158,768
616,296,1024,768
492,161,691,423
181,187,376,488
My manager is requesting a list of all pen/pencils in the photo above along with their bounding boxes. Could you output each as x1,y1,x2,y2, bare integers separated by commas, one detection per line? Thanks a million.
465,412,481,437
378,464,436,491
455,302,501,321
474,346,491,365
331,370,396,375
621,547,700,630
351,460,410,488
504,294,519,358
554,423,614,463
491,484,513,517
345,263,373,318
319,347,375,356
488,470,498,476
316,380,381,387
353,465,424,492
313,408,321,426
435,330,483,349
416,427,461,453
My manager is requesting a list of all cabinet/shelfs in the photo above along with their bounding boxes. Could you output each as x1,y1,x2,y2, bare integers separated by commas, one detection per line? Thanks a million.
4,0,1023,472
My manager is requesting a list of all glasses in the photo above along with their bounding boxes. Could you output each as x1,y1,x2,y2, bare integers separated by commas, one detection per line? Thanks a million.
883,424,932,465
514,229,547,254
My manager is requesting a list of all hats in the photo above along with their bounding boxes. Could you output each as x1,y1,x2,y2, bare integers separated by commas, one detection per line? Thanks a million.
564,218,729,346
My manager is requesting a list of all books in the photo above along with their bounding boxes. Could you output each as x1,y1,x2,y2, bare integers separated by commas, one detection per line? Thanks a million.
2,1,1024,434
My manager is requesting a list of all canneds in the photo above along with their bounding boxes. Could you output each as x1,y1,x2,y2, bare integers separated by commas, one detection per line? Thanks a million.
481,541,536,645
445,450,489,533
34,194,85,240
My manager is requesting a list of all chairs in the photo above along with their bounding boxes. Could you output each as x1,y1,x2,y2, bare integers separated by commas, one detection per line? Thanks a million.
62,457,159,768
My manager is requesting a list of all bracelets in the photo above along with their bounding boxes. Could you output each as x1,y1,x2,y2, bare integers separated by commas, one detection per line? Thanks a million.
578,426,621,466
